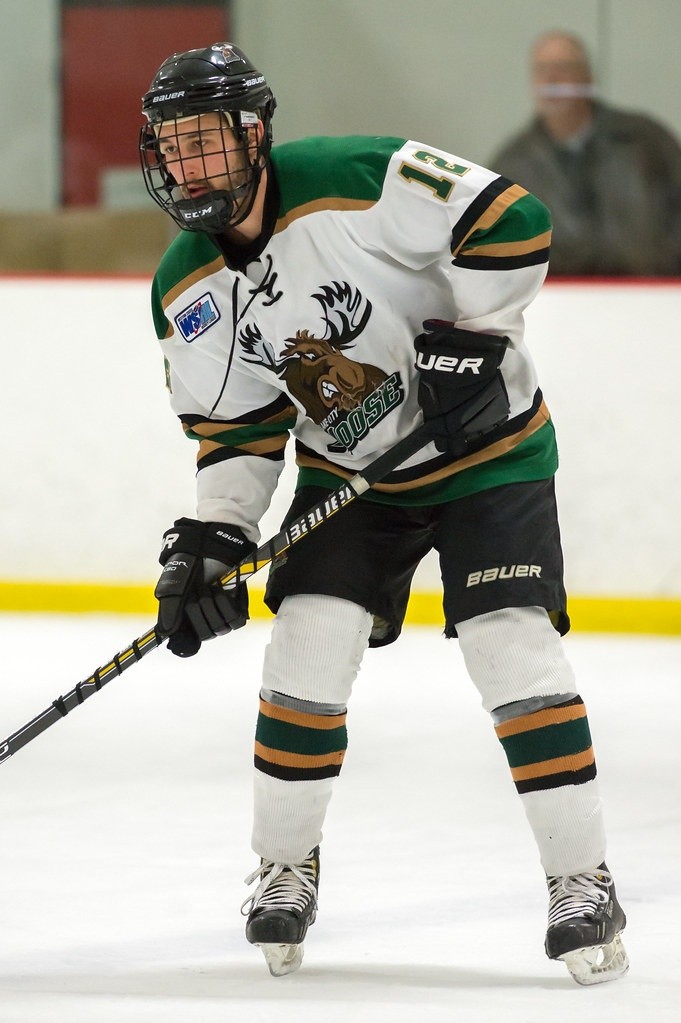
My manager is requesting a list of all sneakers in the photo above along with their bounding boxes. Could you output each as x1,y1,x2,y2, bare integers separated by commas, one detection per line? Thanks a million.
239,845,321,977
543,862,629,986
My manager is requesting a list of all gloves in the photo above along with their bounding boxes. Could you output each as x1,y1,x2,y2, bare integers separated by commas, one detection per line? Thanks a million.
153,517,256,658
413,319,511,456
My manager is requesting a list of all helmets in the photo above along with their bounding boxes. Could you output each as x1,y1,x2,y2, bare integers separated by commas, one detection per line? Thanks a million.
136,40,277,232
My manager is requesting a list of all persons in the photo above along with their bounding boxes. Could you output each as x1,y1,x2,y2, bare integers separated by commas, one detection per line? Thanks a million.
485,32,681,276
138,42,626,958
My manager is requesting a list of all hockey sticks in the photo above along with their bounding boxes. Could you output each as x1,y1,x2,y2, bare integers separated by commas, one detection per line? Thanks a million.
0,422,435,767
